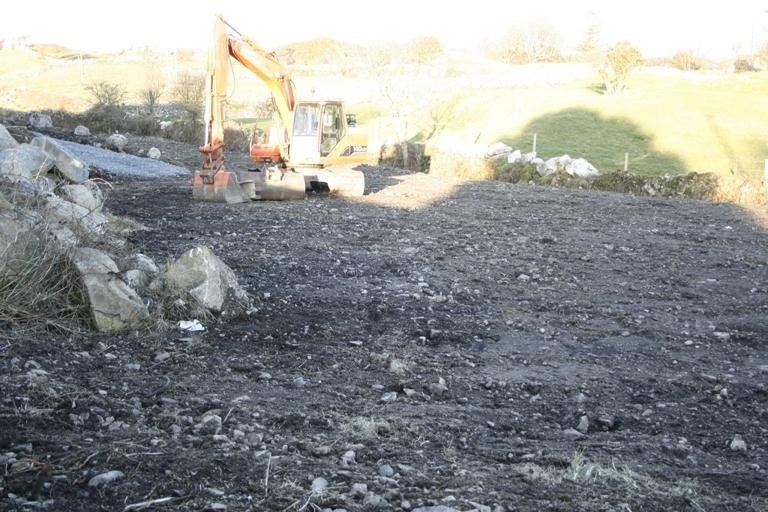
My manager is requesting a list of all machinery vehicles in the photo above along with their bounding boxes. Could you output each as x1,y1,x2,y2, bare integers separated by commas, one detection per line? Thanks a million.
191,15,366,209
